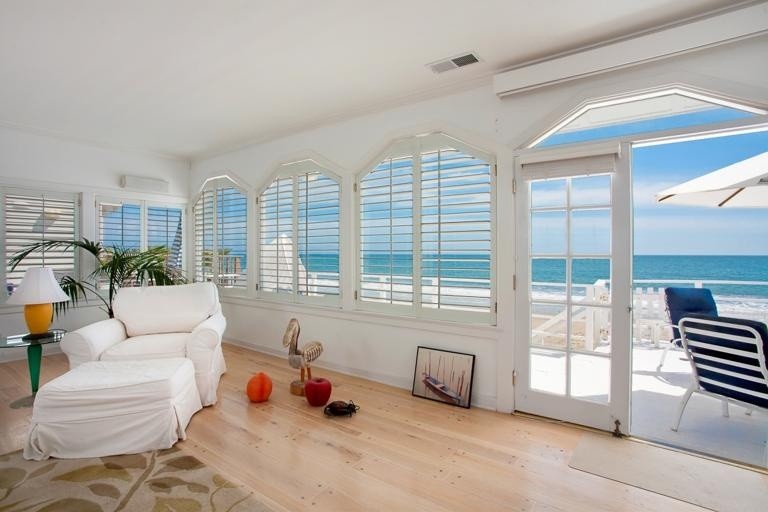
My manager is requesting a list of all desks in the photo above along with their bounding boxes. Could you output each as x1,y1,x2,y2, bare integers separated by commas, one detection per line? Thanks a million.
0,328,67,395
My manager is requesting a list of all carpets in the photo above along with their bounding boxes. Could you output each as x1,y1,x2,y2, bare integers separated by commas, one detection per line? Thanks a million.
0,388,295,511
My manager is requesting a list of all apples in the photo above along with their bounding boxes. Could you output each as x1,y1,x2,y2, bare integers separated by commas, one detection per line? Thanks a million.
305,378,331,407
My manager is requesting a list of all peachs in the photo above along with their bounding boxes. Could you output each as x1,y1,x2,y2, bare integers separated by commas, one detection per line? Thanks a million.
246,373,273,403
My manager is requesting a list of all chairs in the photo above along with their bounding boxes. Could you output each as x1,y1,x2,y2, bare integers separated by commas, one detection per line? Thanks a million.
61,282,227,409
669,312,768,433
654,286,719,382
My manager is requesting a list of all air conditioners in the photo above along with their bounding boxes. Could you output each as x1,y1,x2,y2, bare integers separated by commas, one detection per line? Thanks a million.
121,174,171,196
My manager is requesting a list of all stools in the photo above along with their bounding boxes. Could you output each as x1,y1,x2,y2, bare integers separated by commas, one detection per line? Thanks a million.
23,357,205,462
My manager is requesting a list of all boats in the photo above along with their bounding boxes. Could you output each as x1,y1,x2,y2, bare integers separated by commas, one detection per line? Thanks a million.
425,376,462,400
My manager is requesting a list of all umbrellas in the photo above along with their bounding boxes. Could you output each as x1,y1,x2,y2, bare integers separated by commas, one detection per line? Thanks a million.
653,151,768,209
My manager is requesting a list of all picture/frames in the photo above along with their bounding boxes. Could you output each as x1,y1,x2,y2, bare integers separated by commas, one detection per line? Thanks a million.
410,345,476,409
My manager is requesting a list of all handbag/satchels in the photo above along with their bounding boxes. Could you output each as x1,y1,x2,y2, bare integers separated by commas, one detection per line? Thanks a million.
324,400,360,416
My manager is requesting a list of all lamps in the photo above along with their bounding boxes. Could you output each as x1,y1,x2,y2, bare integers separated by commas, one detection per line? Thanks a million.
6,266,70,335
